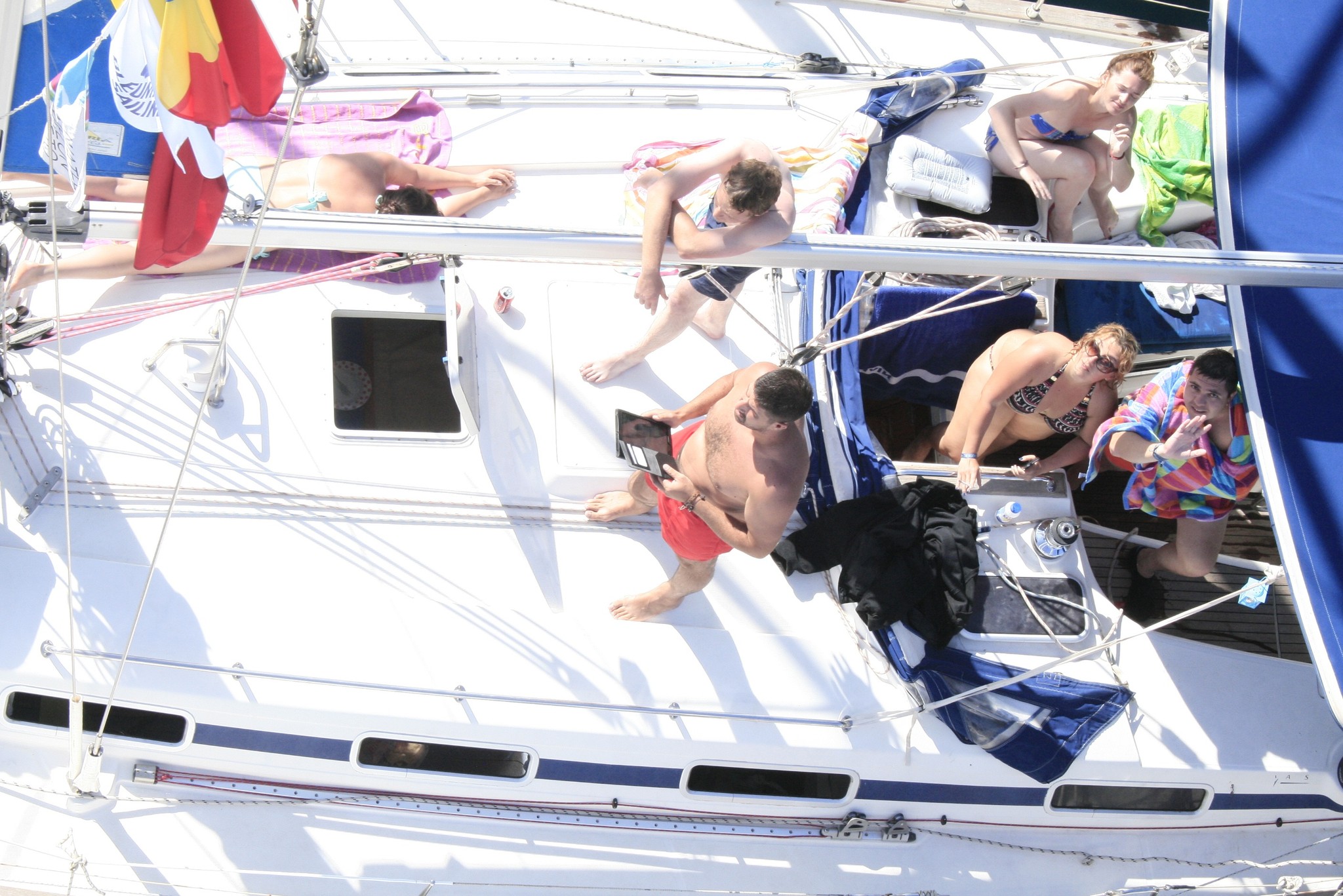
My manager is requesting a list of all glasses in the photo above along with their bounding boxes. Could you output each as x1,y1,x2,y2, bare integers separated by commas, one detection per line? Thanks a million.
1085,338,1119,374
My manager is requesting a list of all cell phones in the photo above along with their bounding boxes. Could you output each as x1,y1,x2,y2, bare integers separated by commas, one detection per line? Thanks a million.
1005,457,1040,475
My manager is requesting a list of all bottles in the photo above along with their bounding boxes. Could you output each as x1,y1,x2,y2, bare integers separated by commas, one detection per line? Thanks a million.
995,501,1022,524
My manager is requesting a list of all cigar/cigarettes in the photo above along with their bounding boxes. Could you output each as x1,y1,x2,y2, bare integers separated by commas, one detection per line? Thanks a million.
958,480,975,488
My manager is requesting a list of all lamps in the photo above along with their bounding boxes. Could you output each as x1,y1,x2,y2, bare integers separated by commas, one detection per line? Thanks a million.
1031,516,1081,560
1238,575,1270,609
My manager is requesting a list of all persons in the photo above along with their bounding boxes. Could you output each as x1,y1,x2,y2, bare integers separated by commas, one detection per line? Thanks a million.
984,42,1157,243
580,135,795,383
584,362,814,622
0,150,517,300
1081,349,1260,621
900,322,1138,496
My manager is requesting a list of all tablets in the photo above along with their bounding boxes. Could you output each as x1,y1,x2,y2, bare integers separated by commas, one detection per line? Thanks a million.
615,409,672,458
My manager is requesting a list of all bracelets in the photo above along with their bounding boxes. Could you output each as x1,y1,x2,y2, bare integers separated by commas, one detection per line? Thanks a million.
1110,149,1126,160
1152,443,1168,461
679,491,706,512
960,453,977,458
1014,160,1028,169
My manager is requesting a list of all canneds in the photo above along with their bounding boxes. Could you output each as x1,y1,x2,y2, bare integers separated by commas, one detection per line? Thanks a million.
494,287,514,314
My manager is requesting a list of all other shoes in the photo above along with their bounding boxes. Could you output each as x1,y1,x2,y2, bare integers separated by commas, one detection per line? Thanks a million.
1127,545,1156,622
